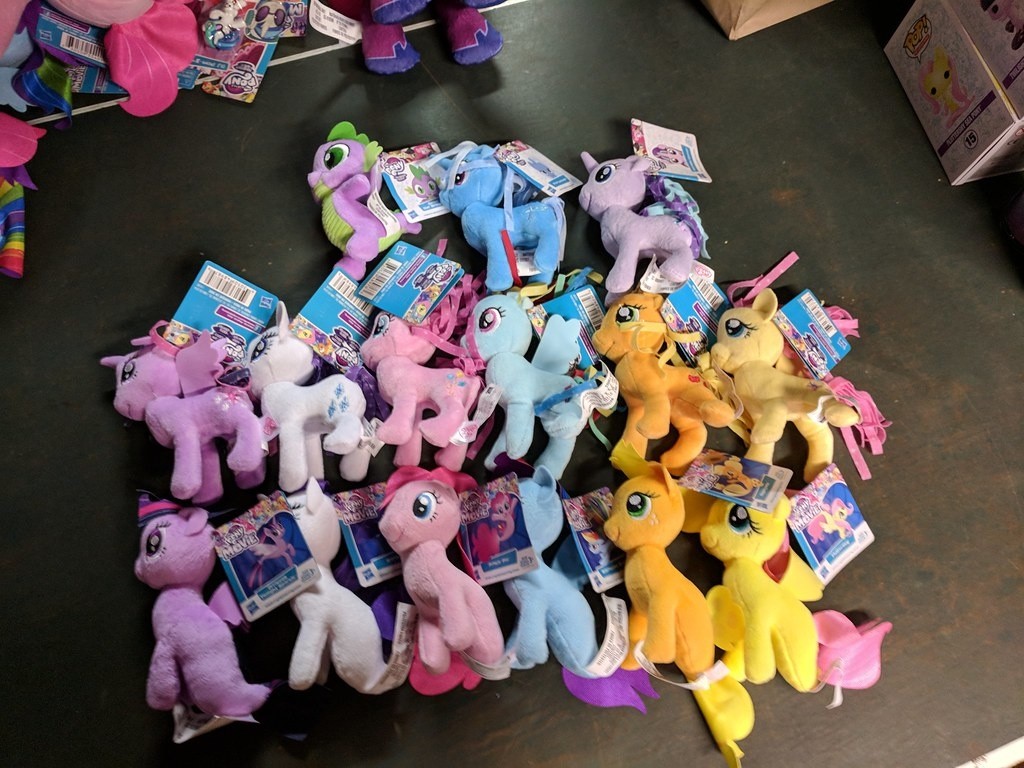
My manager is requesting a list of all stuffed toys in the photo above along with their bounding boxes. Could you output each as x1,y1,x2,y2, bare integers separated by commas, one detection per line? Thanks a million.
0,0,504,113
99,121,893,768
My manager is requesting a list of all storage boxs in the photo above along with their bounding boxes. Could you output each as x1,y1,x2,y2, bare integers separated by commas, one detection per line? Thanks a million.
701,0,834,41
884,0,1024,187
